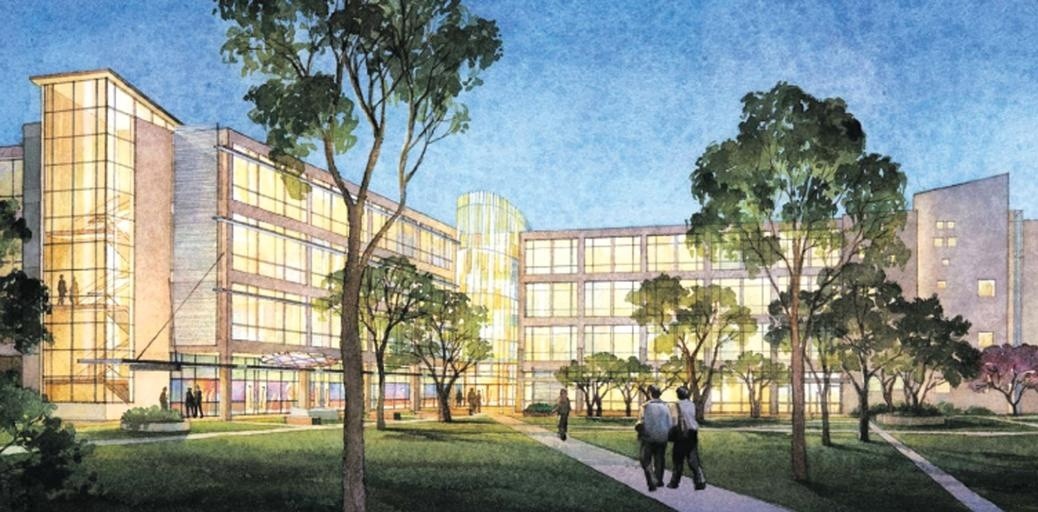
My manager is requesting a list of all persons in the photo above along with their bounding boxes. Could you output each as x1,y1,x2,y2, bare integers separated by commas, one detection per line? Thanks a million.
554,388,570,441
454,389,485,416
69,274,80,305
56,273,67,306
666,387,707,491
637,385,672,492
158,382,203,419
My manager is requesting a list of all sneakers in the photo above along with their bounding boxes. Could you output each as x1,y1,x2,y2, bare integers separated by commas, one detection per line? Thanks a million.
657,483,665,486
695,478,705,490
648,486,656,491
667,482,679,489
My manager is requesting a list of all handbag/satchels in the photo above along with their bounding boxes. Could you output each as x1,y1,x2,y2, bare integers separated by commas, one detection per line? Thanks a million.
668,403,687,443
